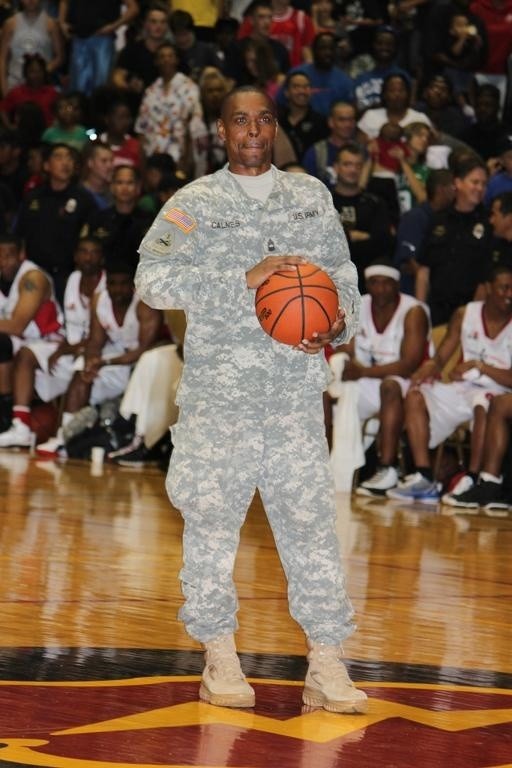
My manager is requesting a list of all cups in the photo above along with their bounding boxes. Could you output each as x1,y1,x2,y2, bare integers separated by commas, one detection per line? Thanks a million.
91,447,105,464
90,463,105,477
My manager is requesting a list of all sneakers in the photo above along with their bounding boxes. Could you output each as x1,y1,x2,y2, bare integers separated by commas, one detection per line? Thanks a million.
356,466,512,509
1,417,33,448
36,425,167,468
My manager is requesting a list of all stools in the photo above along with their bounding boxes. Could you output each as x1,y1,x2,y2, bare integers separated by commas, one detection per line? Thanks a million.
434,418,473,480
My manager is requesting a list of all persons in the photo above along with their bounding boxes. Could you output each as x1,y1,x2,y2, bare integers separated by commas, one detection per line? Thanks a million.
134,85,369,715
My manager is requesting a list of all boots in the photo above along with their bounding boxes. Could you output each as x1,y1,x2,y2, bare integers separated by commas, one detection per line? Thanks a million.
302,642,370,715
199,633,256,706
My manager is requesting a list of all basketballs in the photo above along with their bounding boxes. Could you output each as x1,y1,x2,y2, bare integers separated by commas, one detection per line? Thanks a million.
255,262,339,346
